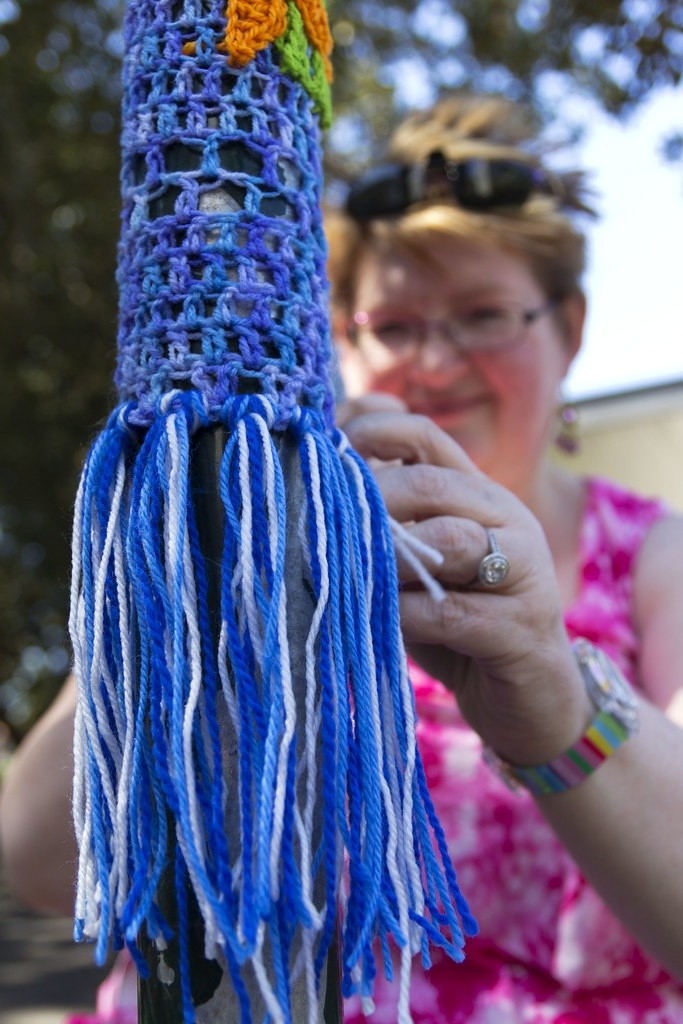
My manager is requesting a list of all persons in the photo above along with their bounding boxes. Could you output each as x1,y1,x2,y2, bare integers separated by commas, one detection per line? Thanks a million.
1,97,681,1023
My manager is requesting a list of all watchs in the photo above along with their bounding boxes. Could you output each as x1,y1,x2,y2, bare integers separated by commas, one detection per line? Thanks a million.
480,636,640,797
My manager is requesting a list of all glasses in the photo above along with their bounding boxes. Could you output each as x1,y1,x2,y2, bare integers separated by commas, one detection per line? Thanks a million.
341,299,560,349
338,147,558,219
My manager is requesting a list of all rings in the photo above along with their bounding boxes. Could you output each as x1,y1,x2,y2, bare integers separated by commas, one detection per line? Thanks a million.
466,527,510,594
